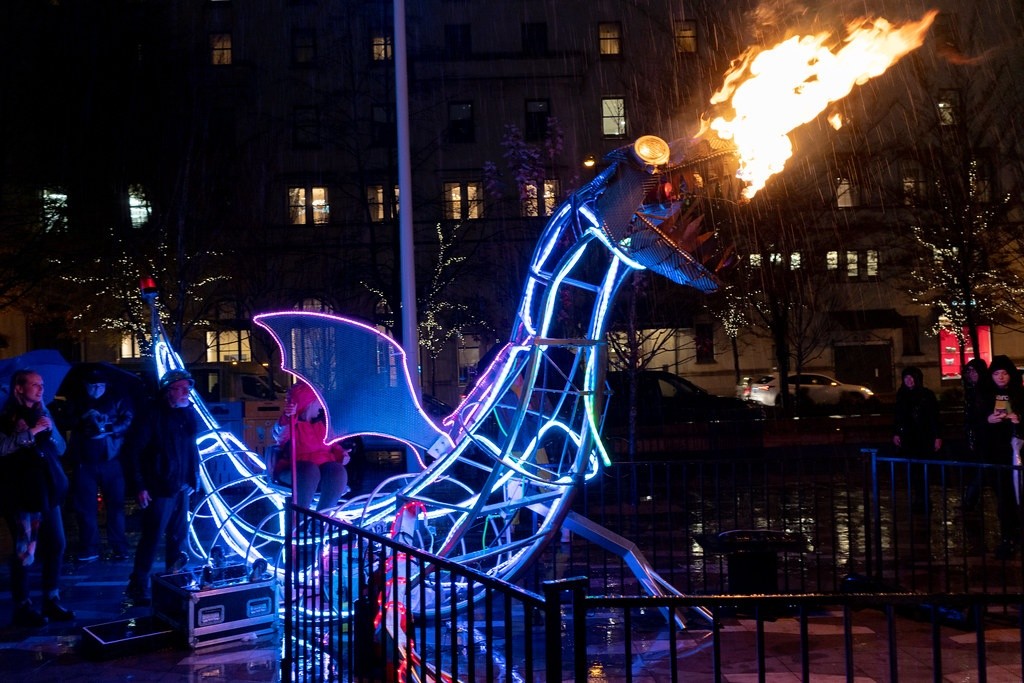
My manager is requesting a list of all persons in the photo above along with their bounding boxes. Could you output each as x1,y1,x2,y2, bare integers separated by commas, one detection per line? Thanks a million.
958,358,988,462
984,355,1024,489
0,369,77,624
122,369,202,602
70,375,135,563
285,381,350,523
887,365,943,491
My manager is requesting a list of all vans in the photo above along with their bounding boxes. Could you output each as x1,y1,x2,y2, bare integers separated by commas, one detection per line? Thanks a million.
102,359,294,451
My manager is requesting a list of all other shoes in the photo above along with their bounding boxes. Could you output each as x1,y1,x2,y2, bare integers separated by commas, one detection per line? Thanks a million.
105,546,130,561
996,536,1015,559
75,546,100,561
41,599,77,623
12,605,49,626
125,581,151,604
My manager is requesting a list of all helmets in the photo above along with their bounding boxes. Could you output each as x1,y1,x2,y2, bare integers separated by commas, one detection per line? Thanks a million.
158,367,193,396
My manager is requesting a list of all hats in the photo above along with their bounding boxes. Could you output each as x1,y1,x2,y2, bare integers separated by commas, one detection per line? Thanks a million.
81,363,111,384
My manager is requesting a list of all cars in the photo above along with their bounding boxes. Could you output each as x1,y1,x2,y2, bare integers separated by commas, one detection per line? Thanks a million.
609,369,766,432
736,372,870,415
